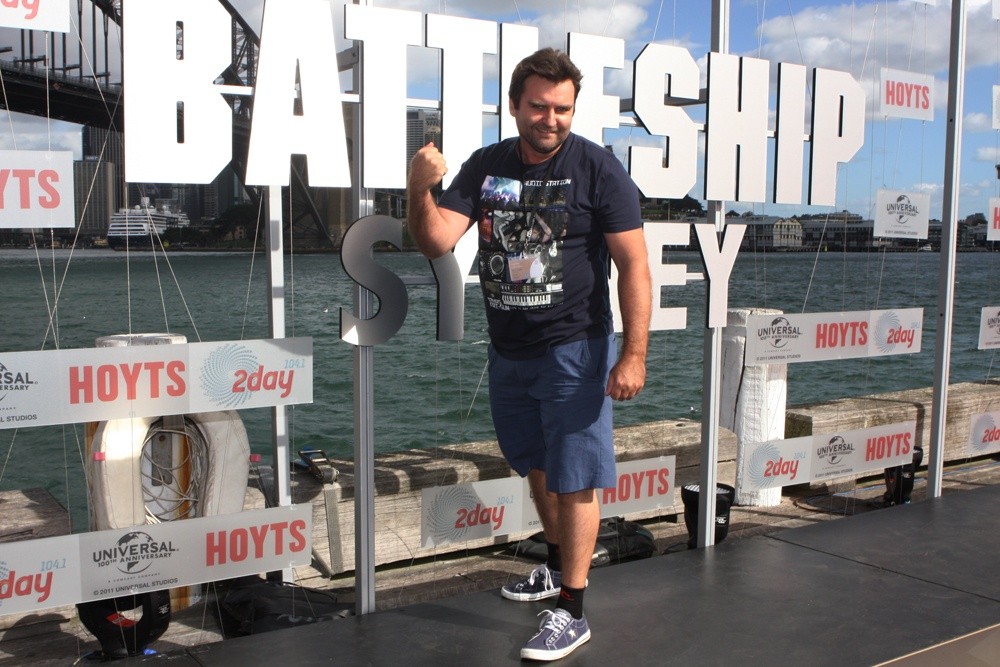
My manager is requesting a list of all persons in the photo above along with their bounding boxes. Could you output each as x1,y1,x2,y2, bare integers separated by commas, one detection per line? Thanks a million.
404,45,653,659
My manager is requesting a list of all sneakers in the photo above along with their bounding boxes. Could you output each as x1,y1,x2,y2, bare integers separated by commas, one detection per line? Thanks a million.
520,607,591,660
501,561,588,601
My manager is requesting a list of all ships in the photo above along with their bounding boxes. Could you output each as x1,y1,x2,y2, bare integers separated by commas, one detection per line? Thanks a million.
106,197,190,251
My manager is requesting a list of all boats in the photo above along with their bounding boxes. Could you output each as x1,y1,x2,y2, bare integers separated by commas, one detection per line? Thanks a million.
919,244,933,252
